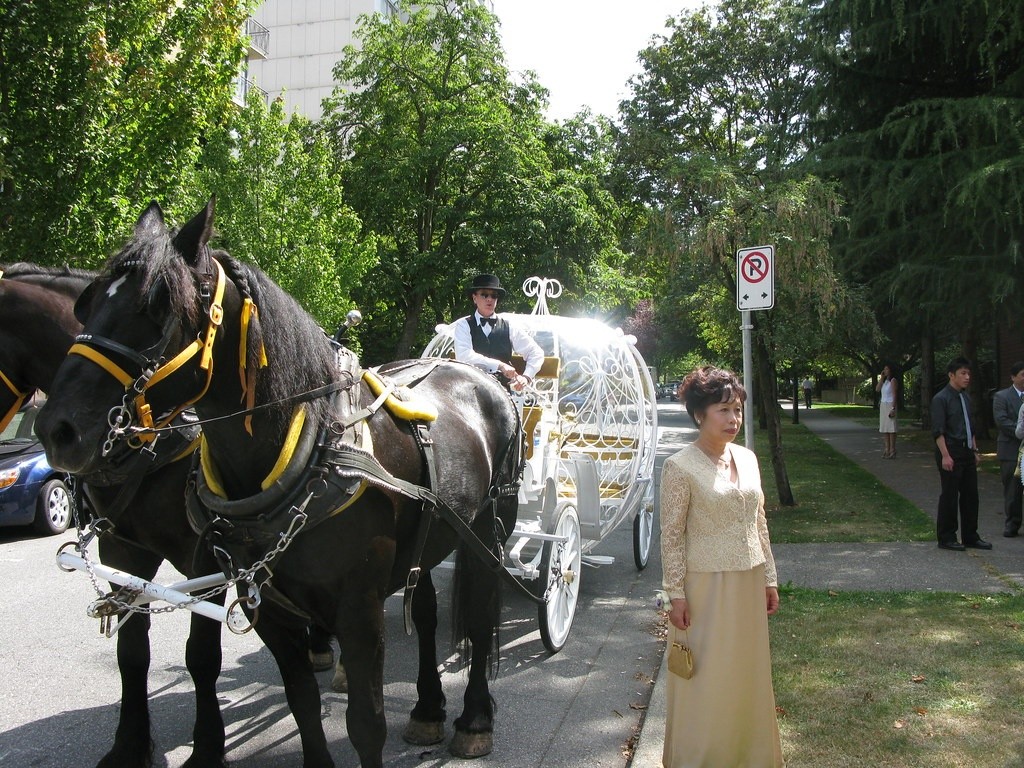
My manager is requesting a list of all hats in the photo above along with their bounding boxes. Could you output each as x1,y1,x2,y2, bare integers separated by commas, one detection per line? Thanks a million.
467,274,506,296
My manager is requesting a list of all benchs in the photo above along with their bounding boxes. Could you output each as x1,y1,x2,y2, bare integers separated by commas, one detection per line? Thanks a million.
556,434,635,499
513,356,560,458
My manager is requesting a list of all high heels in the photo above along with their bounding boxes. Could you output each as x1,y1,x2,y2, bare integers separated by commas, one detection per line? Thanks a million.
880,449,896,459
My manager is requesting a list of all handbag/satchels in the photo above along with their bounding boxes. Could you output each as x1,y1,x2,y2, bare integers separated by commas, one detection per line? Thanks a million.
1014,439,1024,477
667,626,695,680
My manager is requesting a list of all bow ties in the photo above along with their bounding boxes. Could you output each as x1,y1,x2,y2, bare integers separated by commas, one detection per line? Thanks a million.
480,318,497,327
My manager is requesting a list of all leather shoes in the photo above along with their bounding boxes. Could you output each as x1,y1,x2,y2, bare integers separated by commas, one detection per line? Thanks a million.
937,542,966,551
962,539,992,549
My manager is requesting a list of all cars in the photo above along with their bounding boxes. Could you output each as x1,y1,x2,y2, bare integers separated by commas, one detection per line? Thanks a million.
656,381,681,398
558,382,610,414
0,434,87,535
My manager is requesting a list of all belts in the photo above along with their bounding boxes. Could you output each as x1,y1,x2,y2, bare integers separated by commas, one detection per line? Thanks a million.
954,441,968,448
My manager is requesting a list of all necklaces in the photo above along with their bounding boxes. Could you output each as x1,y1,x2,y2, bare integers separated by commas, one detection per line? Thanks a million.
698,438,729,467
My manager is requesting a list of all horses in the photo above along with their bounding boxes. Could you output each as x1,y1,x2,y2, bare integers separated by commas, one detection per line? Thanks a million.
32,192,527,768
0,260,348,768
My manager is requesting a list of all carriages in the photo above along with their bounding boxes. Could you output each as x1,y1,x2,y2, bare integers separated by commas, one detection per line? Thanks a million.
0,191,659,768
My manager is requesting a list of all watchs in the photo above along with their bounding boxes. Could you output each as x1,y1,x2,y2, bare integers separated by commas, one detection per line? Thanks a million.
973,448,979,452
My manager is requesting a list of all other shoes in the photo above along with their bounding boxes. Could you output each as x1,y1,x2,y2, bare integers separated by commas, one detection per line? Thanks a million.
1003,519,1018,537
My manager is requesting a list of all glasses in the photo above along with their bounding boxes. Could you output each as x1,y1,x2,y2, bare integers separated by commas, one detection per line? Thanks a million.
479,293,498,299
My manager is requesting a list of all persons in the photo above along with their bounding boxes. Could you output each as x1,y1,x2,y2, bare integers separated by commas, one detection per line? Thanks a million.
930,357,992,551
876,364,898,459
802,375,812,409
993,362,1024,538
654,365,785,768
455,274,545,397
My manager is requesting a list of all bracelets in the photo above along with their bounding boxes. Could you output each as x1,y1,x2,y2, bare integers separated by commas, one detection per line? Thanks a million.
891,406,895,410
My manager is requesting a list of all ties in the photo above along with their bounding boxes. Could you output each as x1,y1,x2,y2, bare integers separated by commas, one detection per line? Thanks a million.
959,392,972,449
1020,393,1024,398
